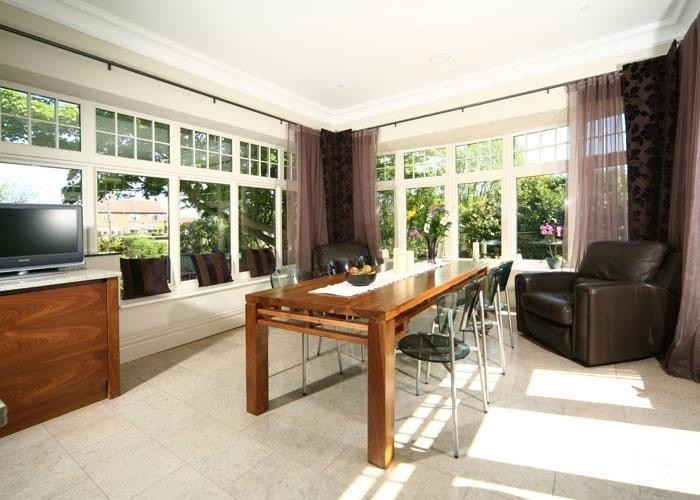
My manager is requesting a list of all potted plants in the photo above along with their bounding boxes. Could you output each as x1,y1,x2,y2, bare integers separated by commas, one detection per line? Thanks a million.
480,220,502,256
459,207,485,260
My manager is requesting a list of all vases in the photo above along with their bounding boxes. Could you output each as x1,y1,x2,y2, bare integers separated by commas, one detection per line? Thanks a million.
426,238,438,262
546,256,563,269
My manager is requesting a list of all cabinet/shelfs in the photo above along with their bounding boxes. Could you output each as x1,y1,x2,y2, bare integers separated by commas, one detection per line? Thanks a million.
0,269,122,439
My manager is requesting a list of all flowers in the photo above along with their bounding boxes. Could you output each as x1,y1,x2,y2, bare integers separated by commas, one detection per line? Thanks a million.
407,201,452,238
540,225,563,257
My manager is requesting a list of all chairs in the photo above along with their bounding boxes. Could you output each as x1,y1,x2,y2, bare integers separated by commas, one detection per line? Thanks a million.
271,243,513,459
515,241,682,367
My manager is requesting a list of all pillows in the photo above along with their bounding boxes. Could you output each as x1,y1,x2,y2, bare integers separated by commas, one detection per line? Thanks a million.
245,248,275,277
190,253,234,287
120,258,172,300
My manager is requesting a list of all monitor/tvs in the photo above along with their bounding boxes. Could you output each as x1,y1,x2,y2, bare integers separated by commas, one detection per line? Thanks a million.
0,203,84,272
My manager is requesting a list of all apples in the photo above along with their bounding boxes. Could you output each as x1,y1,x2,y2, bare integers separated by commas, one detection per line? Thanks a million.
347,264,359,275
363,265,372,273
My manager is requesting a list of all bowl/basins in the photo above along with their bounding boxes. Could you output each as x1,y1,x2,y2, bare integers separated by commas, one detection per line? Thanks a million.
344,270,376,287
